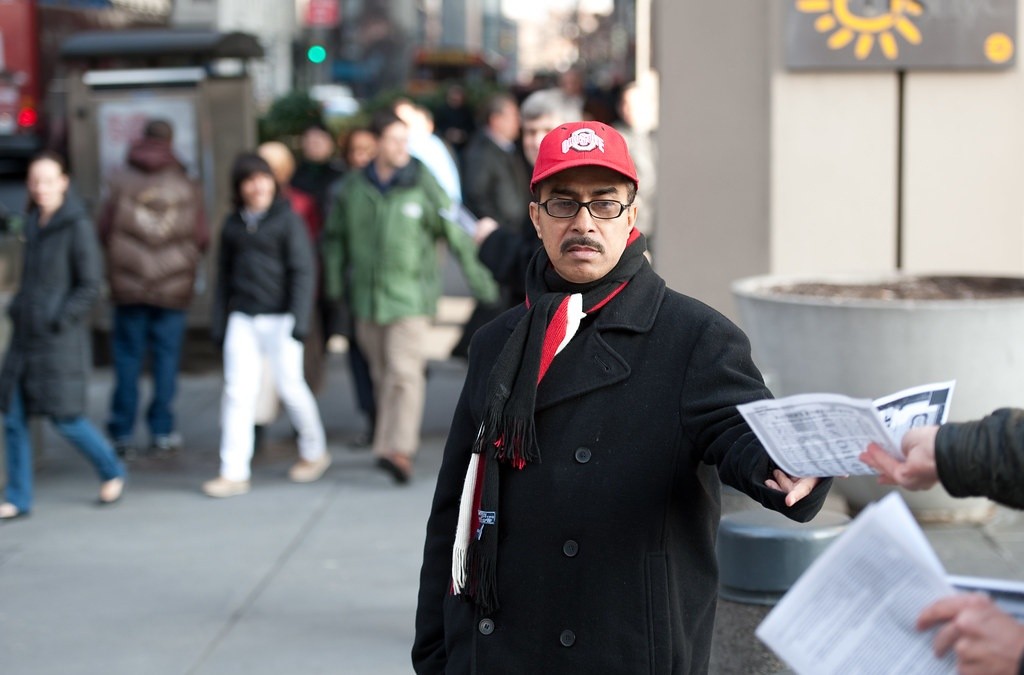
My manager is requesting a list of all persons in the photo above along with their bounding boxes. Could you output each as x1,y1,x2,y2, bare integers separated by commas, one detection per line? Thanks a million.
858,407,1024,675
318,112,501,486
0,152,129,521
201,149,331,496
410,121,832,675
97,120,215,455
0,75,587,521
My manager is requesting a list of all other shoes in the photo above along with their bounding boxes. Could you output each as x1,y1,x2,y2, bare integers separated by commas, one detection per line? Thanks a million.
289,452,333,483
350,411,378,448
376,454,411,483
0,502,24,519
203,476,252,498
153,431,184,449
98,475,125,503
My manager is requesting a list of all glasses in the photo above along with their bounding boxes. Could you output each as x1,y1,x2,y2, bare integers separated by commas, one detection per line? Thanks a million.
538,193,636,218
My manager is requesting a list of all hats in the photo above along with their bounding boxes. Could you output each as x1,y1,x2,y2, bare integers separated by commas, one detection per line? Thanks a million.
530,121,638,199
